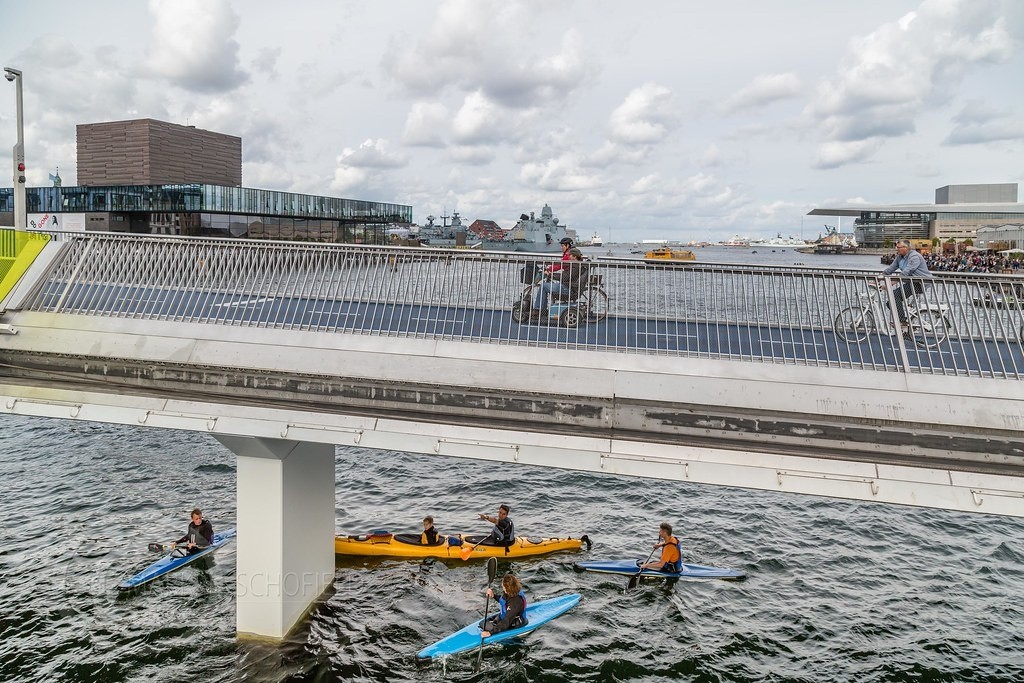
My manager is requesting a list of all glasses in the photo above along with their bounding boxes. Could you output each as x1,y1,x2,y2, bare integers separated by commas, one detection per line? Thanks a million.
896,246,906,249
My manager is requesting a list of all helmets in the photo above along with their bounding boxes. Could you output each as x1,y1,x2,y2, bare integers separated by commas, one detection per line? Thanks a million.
559,238,573,245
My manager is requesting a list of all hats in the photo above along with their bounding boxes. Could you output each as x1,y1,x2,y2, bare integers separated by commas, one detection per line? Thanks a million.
566,249,581,256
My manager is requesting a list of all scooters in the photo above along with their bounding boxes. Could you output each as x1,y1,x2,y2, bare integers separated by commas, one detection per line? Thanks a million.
512,259,592,327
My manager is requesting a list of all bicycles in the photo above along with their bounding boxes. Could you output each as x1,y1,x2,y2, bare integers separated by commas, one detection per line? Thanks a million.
835,275,952,349
520,259,609,323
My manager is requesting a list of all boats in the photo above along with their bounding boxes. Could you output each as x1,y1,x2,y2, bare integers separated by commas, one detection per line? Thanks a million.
724,234,814,248
387,203,577,253
116,529,236,592
335,530,592,561
972,293,1024,310
417,593,585,662
629,240,696,264
573,558,748,581
591,232,603,247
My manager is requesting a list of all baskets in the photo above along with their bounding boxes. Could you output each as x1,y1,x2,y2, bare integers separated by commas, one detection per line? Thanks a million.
520,268,534,285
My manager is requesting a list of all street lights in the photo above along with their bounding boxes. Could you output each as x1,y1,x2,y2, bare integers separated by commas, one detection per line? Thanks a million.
4,66,26,259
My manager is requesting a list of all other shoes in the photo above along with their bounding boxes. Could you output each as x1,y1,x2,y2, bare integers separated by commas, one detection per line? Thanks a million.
524,309,544,317
890,321,907,327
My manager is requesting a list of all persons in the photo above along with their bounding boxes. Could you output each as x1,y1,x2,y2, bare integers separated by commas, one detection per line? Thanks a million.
49,215,59,241
420,516,439,544
477,504,516,543
867,238,1024,327
641,522,684,573
525,238,589,317
481,573,528,638
170,508,214,555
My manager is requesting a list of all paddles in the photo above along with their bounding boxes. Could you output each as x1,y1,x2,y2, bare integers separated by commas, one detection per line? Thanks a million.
472,558,498,679
626,532,662,592
459,530,498,563
147,543,216,557
